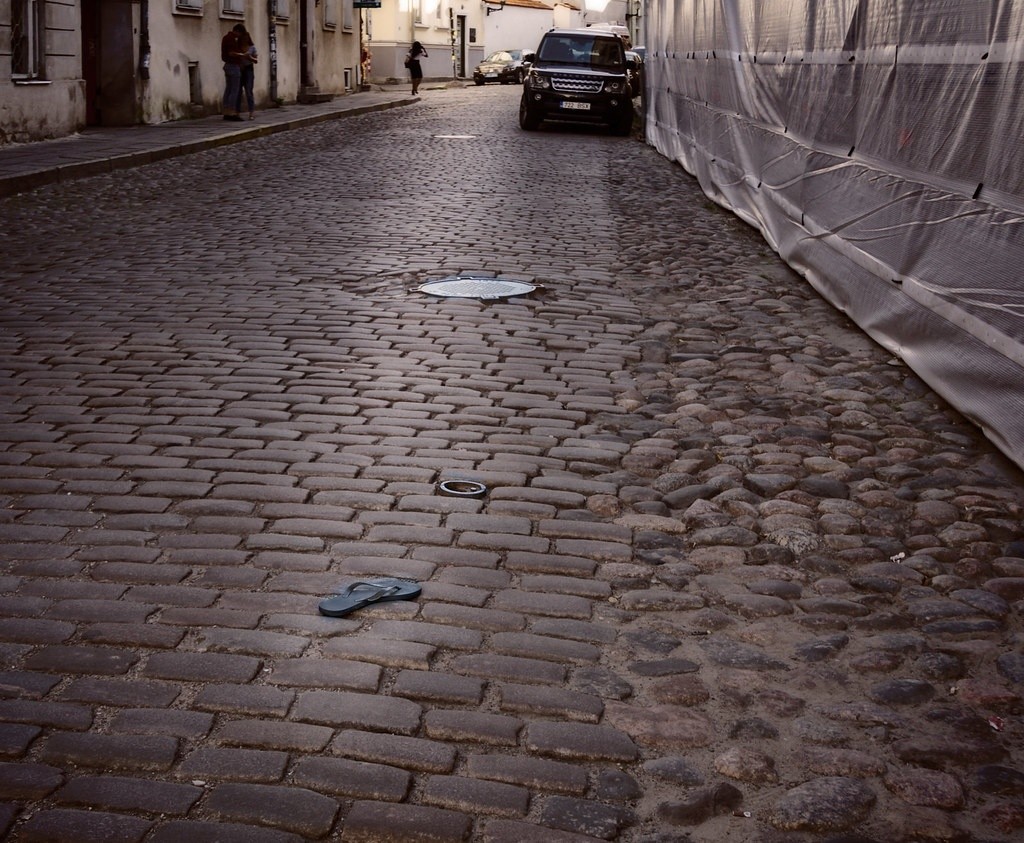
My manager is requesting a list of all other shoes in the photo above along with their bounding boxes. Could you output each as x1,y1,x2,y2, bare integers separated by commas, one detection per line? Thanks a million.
222,114,243,122
249,114,254,120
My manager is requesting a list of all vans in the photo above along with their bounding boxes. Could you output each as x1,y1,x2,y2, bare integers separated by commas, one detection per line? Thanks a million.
585,26,631,45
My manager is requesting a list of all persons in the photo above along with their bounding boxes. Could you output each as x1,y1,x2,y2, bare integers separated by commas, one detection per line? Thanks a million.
405,41,429,96
220,23,258,121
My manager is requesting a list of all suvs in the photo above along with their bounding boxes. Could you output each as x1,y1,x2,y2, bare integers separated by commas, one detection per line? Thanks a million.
518,25,633,136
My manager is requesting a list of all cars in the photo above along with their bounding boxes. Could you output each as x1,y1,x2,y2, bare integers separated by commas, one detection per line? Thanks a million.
473,49,536,85
625,51,641,75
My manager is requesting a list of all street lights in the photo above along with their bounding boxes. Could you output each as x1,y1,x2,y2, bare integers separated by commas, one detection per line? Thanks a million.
625,1,641,21
